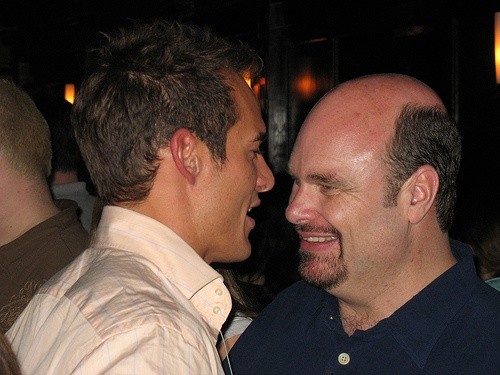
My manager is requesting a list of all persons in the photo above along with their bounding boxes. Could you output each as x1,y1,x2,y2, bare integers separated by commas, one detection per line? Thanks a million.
0,78,90,335
4,20,276,375
221,74,500,375
